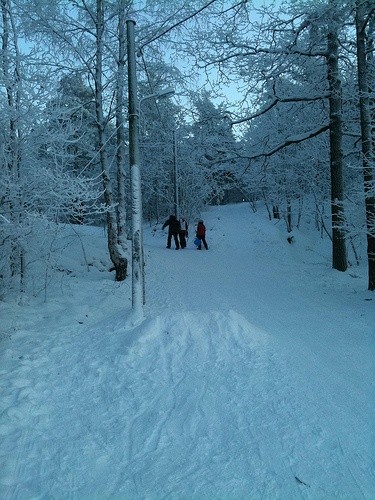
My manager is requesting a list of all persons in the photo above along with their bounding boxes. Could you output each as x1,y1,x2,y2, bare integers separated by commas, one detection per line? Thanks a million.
162,214,180,250
194,220,209,251
178,216,189,250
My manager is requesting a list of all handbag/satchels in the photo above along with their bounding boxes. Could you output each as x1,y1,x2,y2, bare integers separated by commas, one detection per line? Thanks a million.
194,238,202,247
